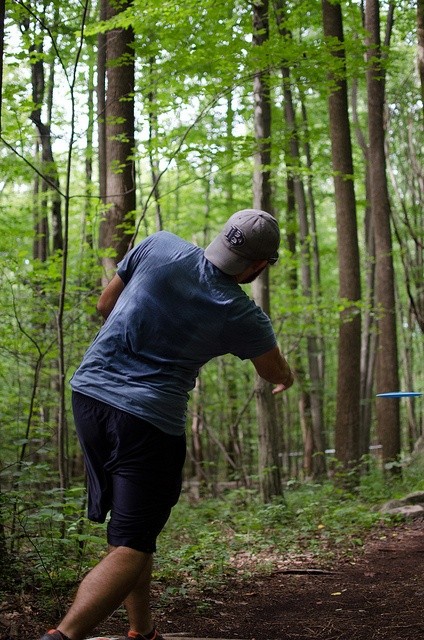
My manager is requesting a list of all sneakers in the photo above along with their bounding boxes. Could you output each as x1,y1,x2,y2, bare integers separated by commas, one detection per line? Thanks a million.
126,628,163,640
38,628,62,640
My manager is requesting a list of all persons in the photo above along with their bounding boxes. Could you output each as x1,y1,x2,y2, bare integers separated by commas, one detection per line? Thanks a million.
39,207,296,640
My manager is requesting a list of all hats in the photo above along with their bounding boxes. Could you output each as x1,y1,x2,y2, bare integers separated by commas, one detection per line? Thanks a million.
203,208,280,276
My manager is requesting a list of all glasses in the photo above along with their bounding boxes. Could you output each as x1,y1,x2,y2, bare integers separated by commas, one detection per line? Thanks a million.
266,252,279,265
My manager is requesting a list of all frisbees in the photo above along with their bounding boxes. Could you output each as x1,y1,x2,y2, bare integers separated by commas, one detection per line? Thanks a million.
376,392,423,398
